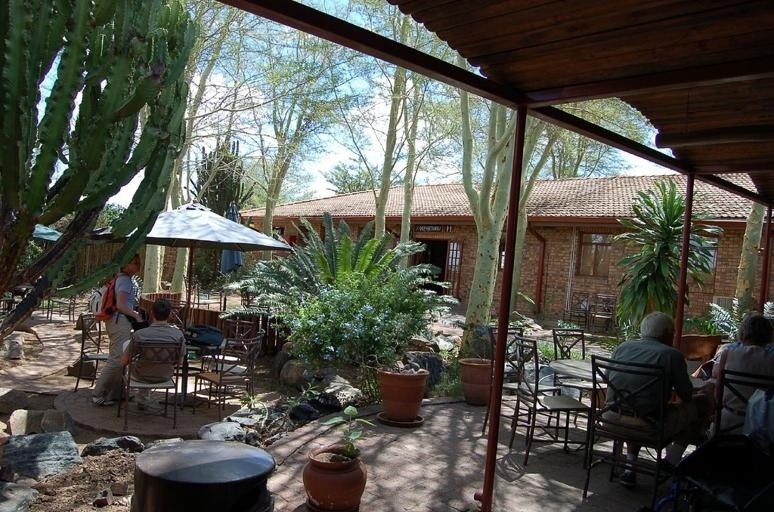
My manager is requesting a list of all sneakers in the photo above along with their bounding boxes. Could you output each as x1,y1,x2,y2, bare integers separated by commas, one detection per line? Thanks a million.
135,401,149,411
615,469,637,487
111,393,136,404
659,458,686,477
90,394,116,407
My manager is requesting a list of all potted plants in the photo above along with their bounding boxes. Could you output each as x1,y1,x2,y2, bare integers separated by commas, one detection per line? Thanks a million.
303,406,374,512
375,336,430,421
452,320,495,406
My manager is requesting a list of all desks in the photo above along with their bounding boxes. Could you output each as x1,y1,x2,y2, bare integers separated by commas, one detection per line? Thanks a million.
550,358,710,408
158,344,222,408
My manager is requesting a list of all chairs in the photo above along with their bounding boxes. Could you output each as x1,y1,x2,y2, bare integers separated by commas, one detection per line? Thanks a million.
47,294,75,323
562,291,590,329
191,338,254,421
200,329,266,393
717,369,774,436
582,355,686,508
75,312,109,392
589,295,616,332
482,326,561,441
547,329,588,426
117,329,184,431
510,337,592,469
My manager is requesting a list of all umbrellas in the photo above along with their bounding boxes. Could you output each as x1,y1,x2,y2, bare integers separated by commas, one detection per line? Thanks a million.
87,201,293,408
30,222,64,244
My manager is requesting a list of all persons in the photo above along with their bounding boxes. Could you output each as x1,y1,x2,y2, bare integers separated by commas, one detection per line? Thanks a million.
92,253,144,406
704,315,774,451
120,300,187,410
604,312,699,487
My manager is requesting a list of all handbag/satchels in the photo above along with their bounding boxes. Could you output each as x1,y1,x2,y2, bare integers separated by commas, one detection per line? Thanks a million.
696,356,719,381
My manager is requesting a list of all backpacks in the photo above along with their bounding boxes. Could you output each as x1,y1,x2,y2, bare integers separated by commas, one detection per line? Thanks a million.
88,274,118,322
